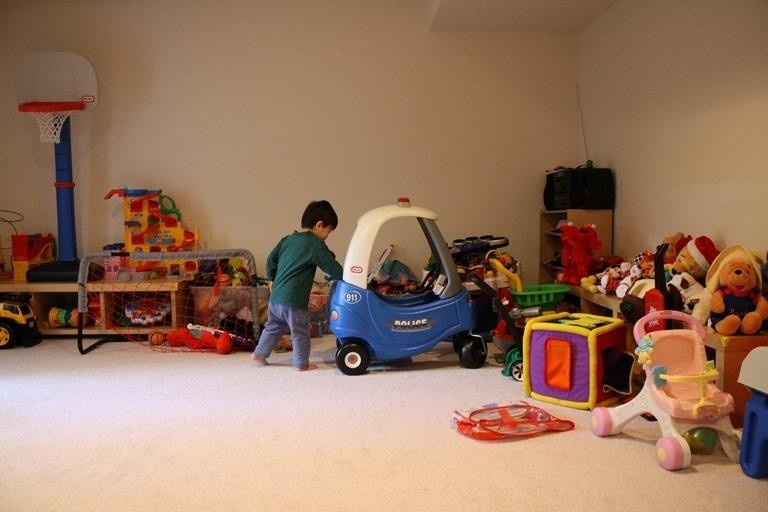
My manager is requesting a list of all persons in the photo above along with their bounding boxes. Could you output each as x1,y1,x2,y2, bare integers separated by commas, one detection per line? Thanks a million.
252,199,343,372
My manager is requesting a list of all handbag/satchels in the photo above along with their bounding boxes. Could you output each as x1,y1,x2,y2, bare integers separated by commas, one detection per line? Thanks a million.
543,166,617,210
447,395,575,443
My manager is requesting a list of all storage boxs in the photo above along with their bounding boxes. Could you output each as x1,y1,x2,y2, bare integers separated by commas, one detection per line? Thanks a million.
520,311,626,410
11,234,54,262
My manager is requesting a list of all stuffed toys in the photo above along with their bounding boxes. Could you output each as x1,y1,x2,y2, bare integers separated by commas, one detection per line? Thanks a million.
165,328,232,353
558,222,706,333
706,243,768,334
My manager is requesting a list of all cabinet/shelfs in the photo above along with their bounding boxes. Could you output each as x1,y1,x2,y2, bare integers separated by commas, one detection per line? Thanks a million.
568,288,768,428
539,208,612,284
0,282,188,339
